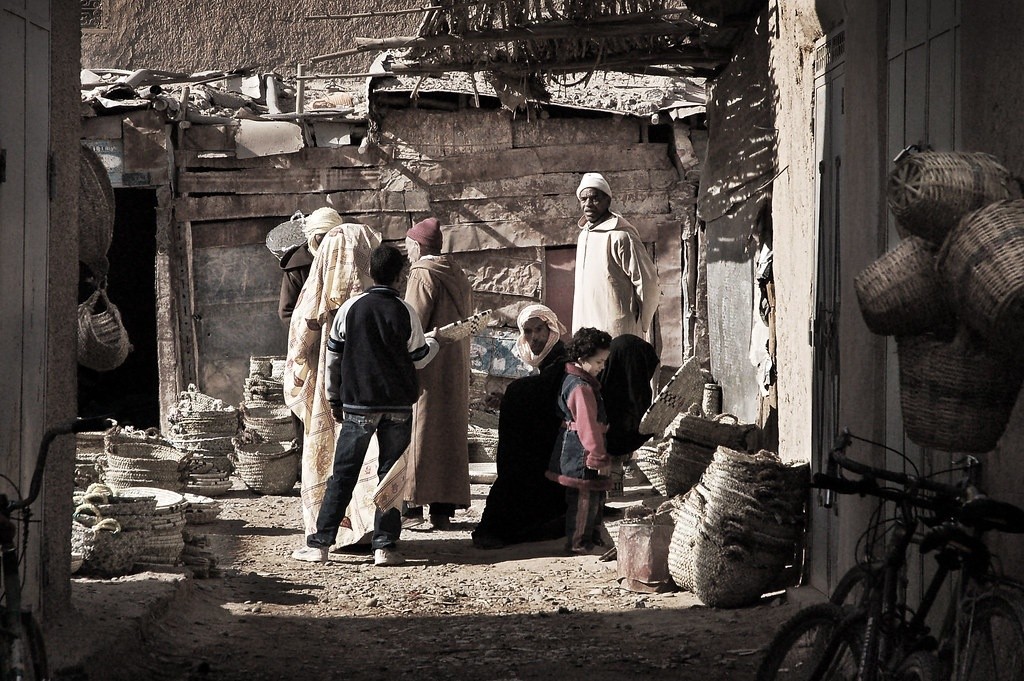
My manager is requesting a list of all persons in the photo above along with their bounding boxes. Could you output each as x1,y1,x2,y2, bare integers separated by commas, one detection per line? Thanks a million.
471,305,661,550
292,244,454,566
278,207,410,553
545,327,616,553
401,217,481,530
571,173,659,340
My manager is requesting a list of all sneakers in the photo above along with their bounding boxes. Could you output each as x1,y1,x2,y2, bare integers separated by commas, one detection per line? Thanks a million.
374,548,405,566
291,546,329,562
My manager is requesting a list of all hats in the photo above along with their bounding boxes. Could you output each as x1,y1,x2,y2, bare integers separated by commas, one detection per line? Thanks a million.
576,172,612,202
406,217,443,249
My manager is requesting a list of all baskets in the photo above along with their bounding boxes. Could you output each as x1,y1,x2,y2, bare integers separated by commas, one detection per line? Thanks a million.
69,356,302,573
265,210,307,262
854,150,1024,454
76,290,134,372
632,356,811,610
